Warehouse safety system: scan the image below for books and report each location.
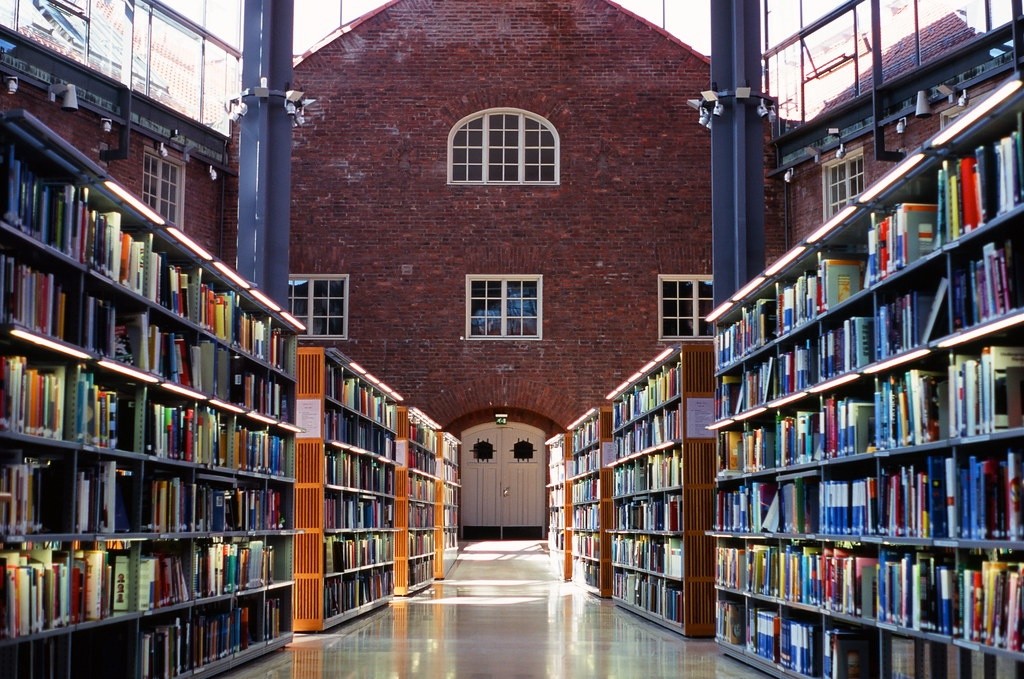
[0,154,460,678]
[548,132,1024,679]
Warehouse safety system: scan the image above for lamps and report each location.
[209,164,217,180]
[915,90,932,118]
[784,168,794,182]
[958,90,968,106]
[6,77,18,95]
[48,84,78,111]
[896,116,907,133]
[100,118,112,133]
[154,141,168,157]
[835,144,846,159]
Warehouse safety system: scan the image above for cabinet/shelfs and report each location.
[293,347,404,632]
[0,109,305,679]
[605,343,716,636]
[544,433,572,581]
[395,406,442,596]
[567,407,613,597]
[707,82,1023,679]
[435,432,462,578]
